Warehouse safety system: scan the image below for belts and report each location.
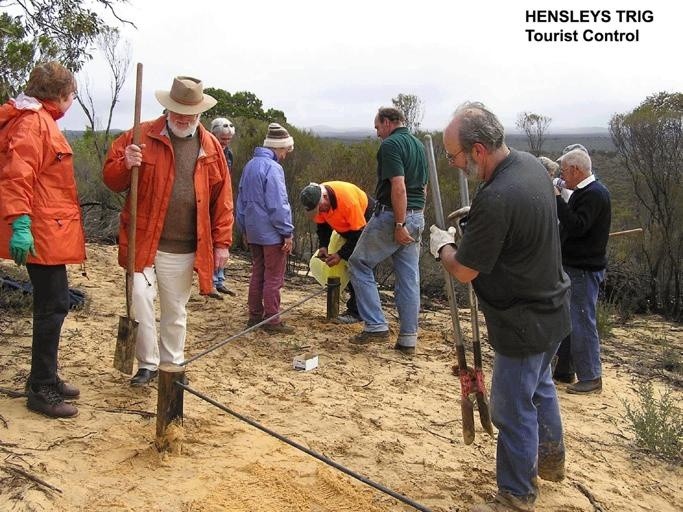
[381,206,422,212]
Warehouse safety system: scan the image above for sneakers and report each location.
[247,320,295,334]
[131,369,158,385]
[335,315,358,323]
[552,374,575,385]
[350,331,389,344]
[210,285,234,300]
[470,492,535,512]
[567,378,603,395]
[26,375,79,418]
[394,343,415,354]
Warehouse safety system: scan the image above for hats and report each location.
[156,76,217,115]
[263,123,294,148]
[301,186,320,218]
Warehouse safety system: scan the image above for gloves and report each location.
[429,225,457,260]
[9,215,37,267]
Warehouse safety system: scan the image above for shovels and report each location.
[113,63,143,375]
[424,134,494,446]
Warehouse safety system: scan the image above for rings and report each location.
[284,243,287,246]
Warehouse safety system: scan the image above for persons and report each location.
[429,100,573,511]
[347,106,429,356]
[300,179,373,324]
[200,117,236,300]
[235,123,297,334]
[537,143,611,396]
[0,61,86,419]
[102,77,235,386]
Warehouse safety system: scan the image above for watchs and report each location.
[394,221,406,228]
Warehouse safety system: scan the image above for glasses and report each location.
[446,149,462,165]
